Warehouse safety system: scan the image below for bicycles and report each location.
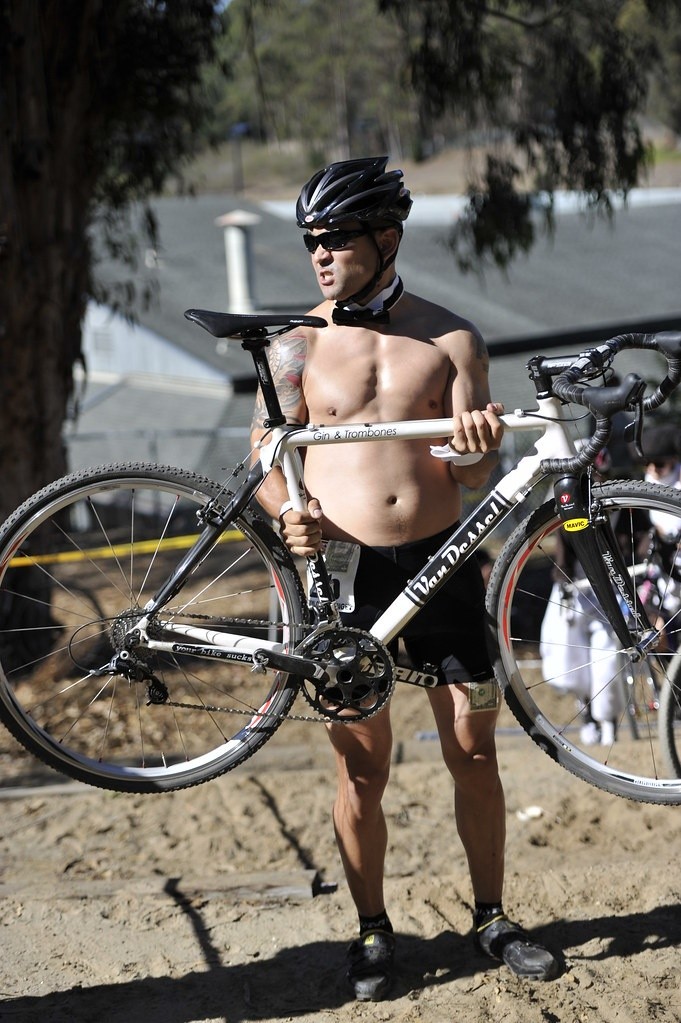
[1,310,681,805]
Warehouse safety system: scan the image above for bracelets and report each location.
[280,501,290,515]
[428,443,484,466]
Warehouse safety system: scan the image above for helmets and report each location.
[296,155,414,228]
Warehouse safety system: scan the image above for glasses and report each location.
[638,458,666,469]
[302,227,369,252]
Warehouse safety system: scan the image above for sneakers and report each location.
[469,911,559,982]
[345,928,400,1001]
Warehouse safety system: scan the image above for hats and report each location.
[626,421,681,457]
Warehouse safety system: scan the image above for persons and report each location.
[249,155,558,1002]
[539,457,681,746]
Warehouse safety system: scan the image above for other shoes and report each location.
[579,720,601,748]
[600,717,618,748]
